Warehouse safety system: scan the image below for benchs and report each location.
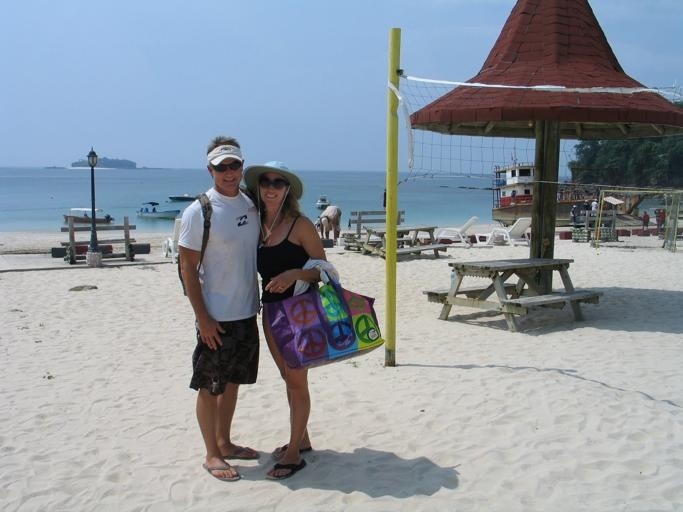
[384,243,448,254]
[505,291,607,332]
[423,283,515,319]
[356,237,413,255]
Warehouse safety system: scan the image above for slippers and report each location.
[202,462,241,481]
[223,445,261,461]
[271,442,312,460]
[265,459,307,480]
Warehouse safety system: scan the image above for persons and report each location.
[177,138,262,482]
[642,209,664,231]
[140,207,157,213]
[243,162,326,480]
[570,198,609,224]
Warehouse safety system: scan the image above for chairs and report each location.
[435,216,480,249]
[476,218,532,248]
[163,218,182,264]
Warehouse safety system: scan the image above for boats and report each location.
[575,213,615,226]
[64,207,113,223]
[316,194,330,209]
[73,158,137,169]
[135,202,180,218]
[168,196,197,200]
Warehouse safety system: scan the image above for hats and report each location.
[206,144,245,167]
[242,160,304,202]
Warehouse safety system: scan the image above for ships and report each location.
[492,161,647,226]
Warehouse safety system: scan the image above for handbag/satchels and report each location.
[266,268,385,369]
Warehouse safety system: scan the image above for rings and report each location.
[279,287,283,289]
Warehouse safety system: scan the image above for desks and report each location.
[362,226,439,257]
[438,259,585,333]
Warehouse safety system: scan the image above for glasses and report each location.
[210,160,242,172]
[257,174,290,190]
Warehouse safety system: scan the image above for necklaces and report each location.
[263,216,285,247]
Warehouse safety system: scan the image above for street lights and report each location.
[87,147,101,253]
